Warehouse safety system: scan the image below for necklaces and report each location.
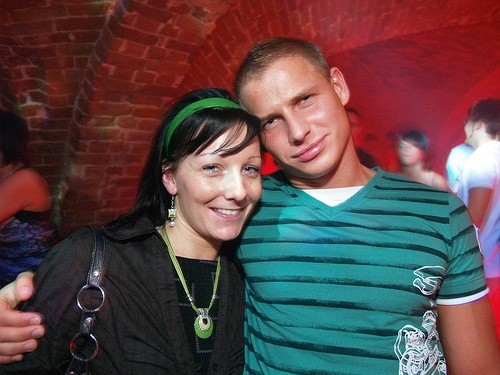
[161,227,222,339]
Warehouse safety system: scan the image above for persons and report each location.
[0,86,266,375]
[345,107,380,172]
[0,110,63,289]
[390,129,450,192]
[0,35,500,375]
[445,98,500,350]
